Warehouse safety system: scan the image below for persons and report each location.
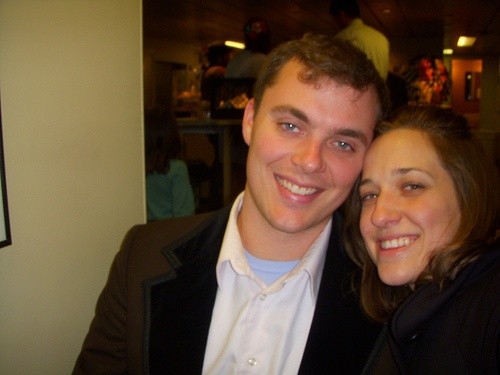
[200,41,233,117]
[145,106,194,221]
[70,36,386,375]
[342,106,500,375]
[221,17,272,119]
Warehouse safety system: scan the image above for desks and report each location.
[176,117,242,205]
